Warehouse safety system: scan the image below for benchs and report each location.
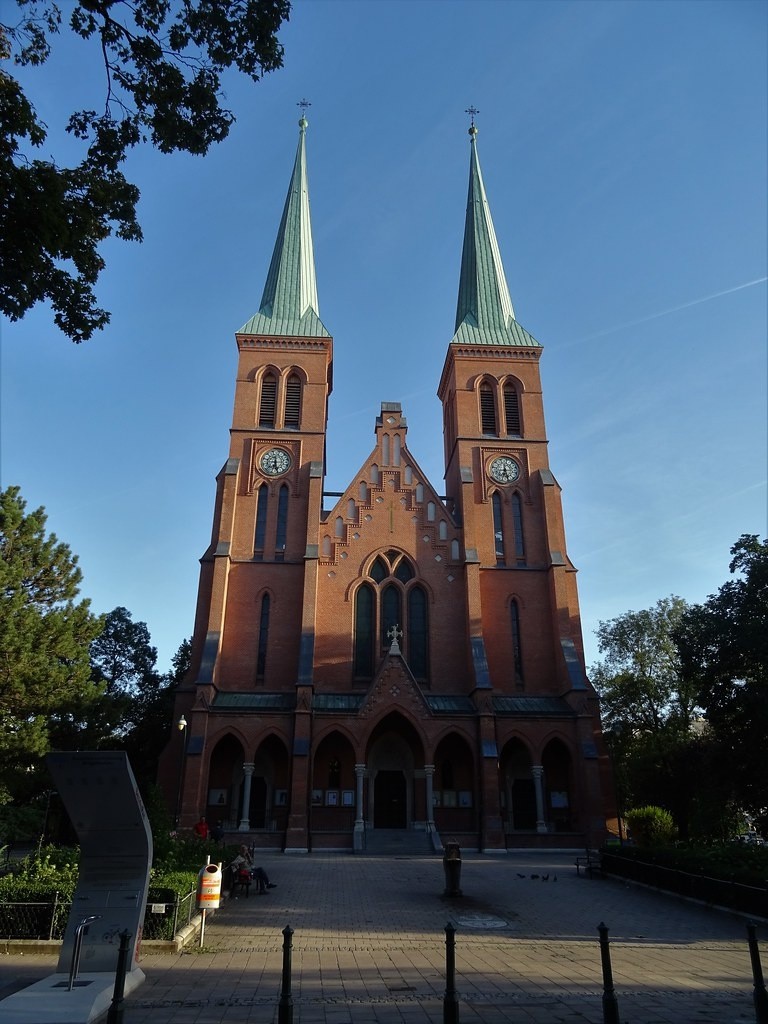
[229,866,258,898]
[575,853,610,880]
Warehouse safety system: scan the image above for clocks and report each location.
[485,451,525,486]
[255,443,296,479]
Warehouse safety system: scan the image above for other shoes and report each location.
[260,890,270,895]
[266,883,277,889]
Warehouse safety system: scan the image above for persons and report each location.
[231,845,277,896]
[193,815,225,846]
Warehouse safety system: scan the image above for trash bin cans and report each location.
[194,863,223,909]
